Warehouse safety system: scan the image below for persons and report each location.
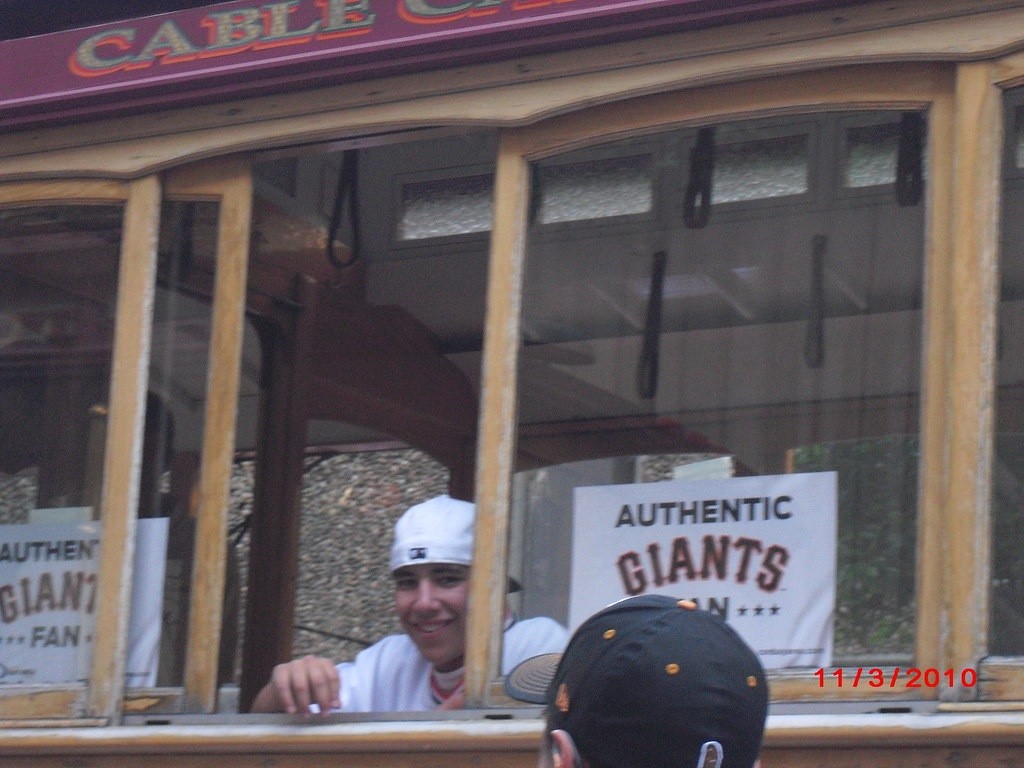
[255,493,570,718]
[504,594,770,768]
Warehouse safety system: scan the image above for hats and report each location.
[387,496,524,594]
[504,594,771,768]
